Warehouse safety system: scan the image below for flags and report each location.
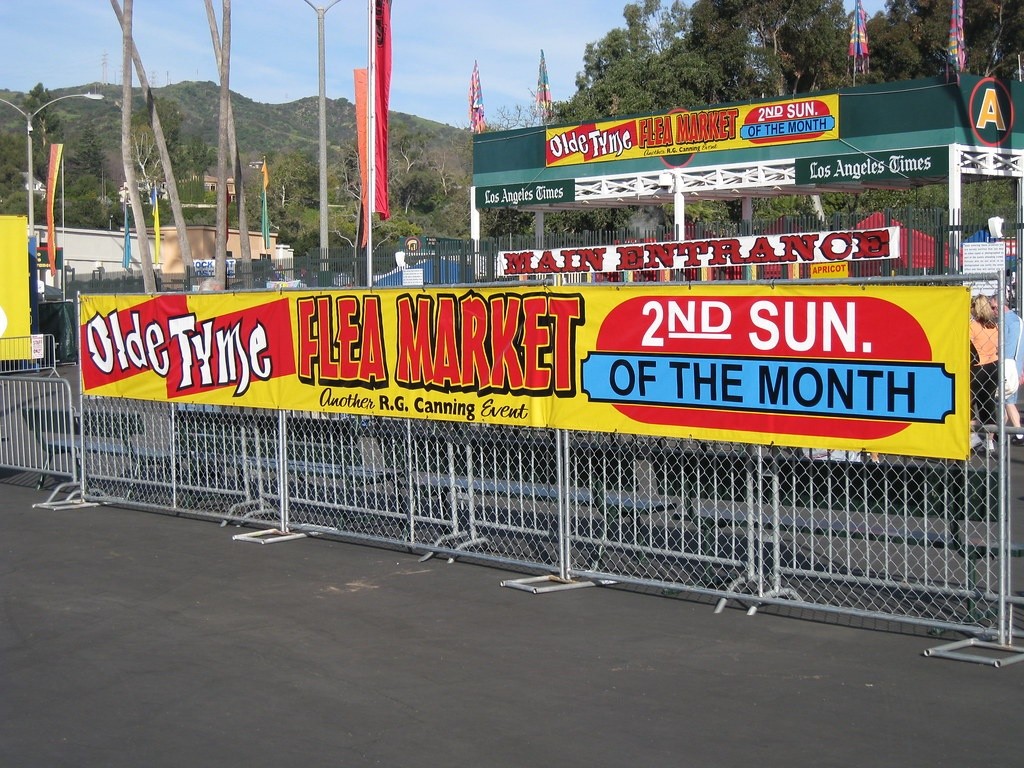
[468,65,485,133]
[946,1,965,87]
[261,161,270,249]
[215,187,231,242]
[372,0,393,221]
[849,1,869,75]
[152,189,160,265]
[536,49,551,119]
[47,144,64,277]
[354,69,369,248]
[122,199,130,270]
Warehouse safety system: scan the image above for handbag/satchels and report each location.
[970,340,980,365]
[994,359,1020,400]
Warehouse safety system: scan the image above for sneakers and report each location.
[973,442,995,453]
[1005,436,1024,446]
[970,432,982,449]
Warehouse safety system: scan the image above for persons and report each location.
[990,295,1024,446]
[970,294,999,453]
